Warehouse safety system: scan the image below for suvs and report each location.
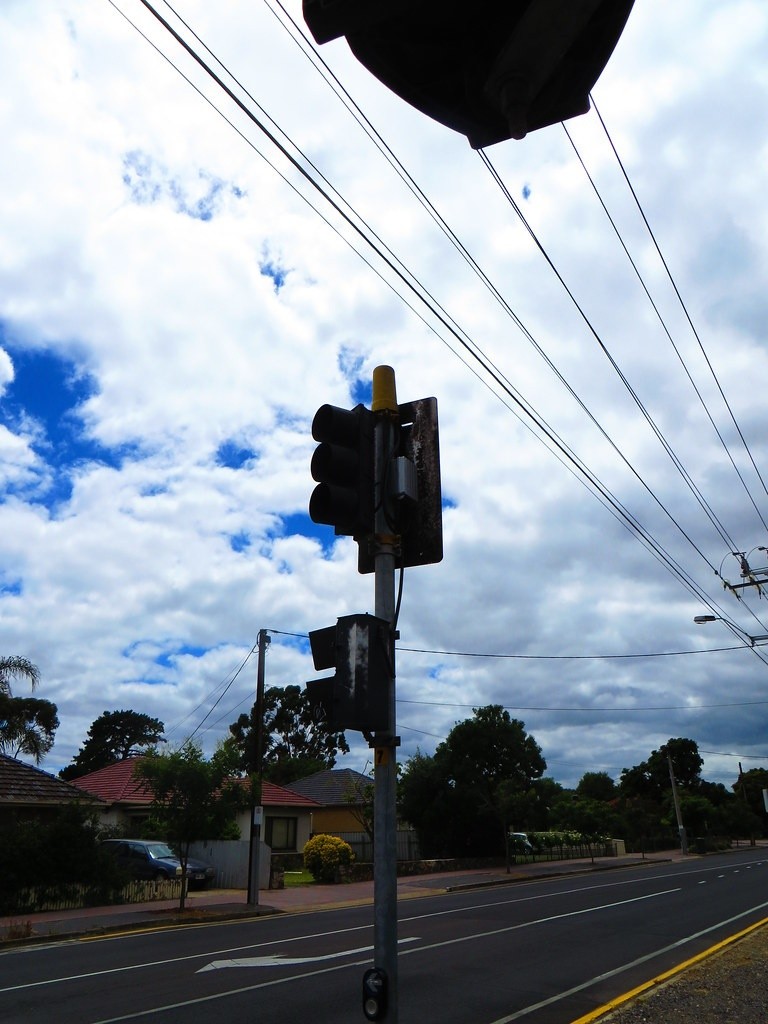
[106,837,217,893]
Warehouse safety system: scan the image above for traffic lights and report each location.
[299,401,369,534]
[304,612,392,736]
